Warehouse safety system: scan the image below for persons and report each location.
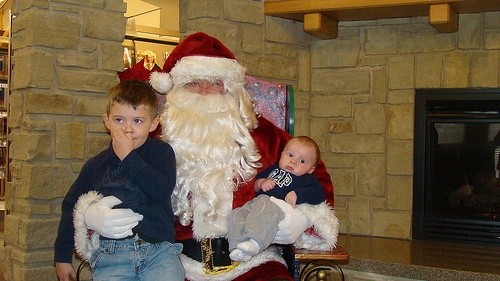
[227,135,320,262]
[73,31,339,281]
[53,80,186,279]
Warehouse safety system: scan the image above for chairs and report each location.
[75,243,351,281]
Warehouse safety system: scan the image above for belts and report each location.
[175,236,234,268]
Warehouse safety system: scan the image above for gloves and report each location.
[270,196,312,245]
[84,196,144,239]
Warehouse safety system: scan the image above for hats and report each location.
[149,31,246,95]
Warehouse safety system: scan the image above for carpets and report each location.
[299,257,500,280]
